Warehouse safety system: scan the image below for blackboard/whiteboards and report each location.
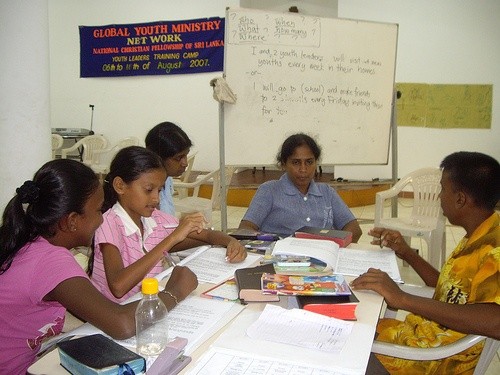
[222,4,399,166]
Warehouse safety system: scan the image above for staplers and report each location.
[146,335,192,375]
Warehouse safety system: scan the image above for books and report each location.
[56,226,403,375]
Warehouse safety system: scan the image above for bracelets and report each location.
[161,290,178,303]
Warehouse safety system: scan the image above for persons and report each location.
[91,146,247,304]
[0,159,198,375]
[238,134,363,243]
[349,150,500,375]
[145,122,206,252]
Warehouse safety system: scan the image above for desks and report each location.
[23,233,403,375]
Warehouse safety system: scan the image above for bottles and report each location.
[135,277,169,361]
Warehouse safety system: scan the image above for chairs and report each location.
[49,132,142,185]
[164,149,237,216]
[375,160,447,279]
[368,284,500,375]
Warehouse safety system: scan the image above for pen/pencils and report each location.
[162,224,179,228]
[163,250,177,267]
